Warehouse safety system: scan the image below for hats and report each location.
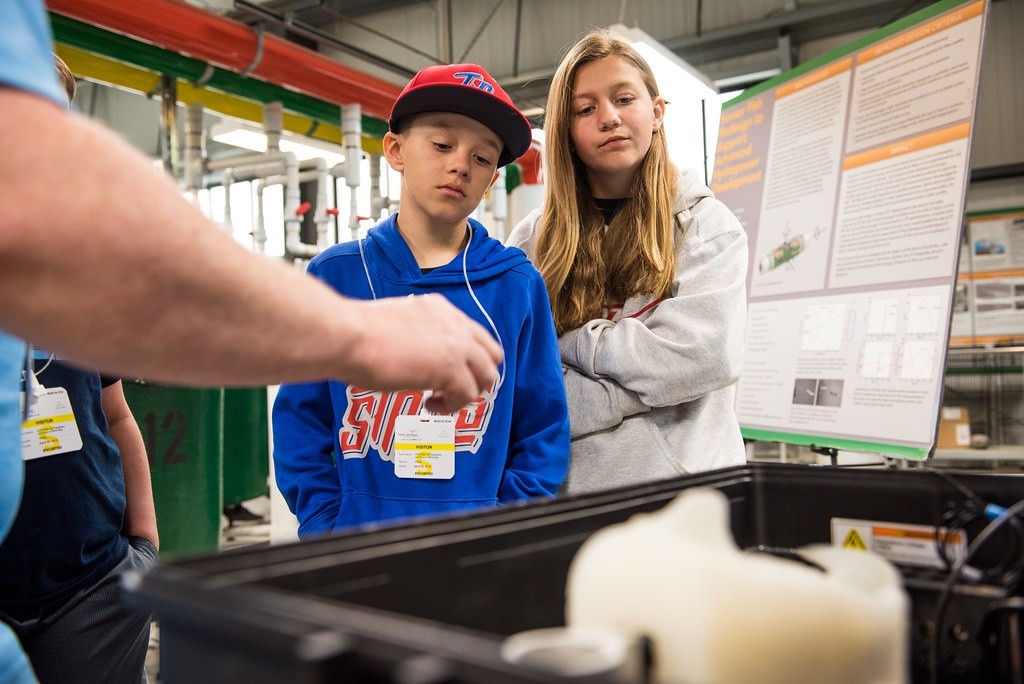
[389,63,532,168]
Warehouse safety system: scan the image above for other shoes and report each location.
[224,505,264,525]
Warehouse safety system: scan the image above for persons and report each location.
[0,0,502,684]
[272,62,571,539]
[503,35,748,492]
[0,343,159,684]
